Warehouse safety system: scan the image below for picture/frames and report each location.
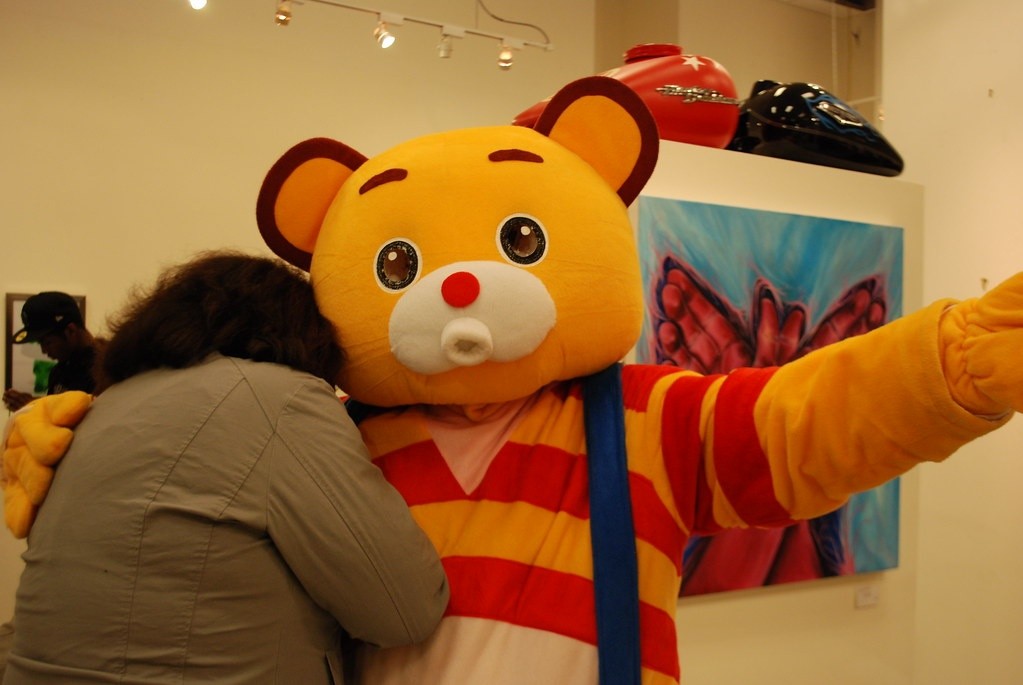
[4,292,87,399]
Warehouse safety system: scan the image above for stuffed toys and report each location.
[1,72,1023,685]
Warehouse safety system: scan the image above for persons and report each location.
[1,252,455,685]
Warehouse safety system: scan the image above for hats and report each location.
[11,291,81,344]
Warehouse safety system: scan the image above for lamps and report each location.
[435,25,466,58]
[272,0,305,27]
[372,12,404,49]
[496,38,524,71]
